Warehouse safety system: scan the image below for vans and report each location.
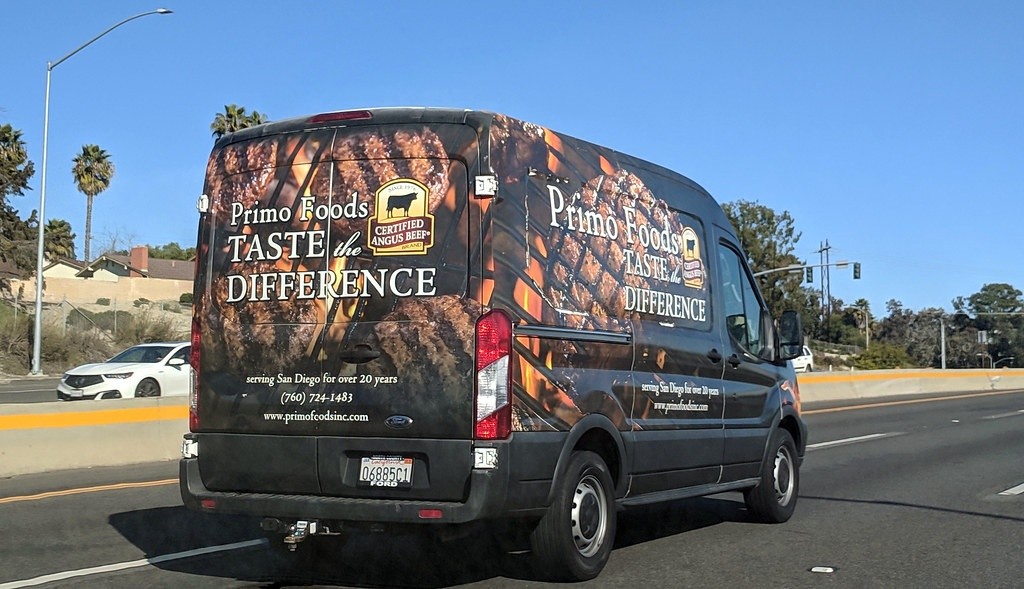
[176,105,810,583]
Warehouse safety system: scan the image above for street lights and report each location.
[993,357,1014,369]
[976,353,992,369]
[841,305,869,353]
[29,9,174,377]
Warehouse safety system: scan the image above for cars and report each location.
[789,345,814,374]
[56,341,193,402]
